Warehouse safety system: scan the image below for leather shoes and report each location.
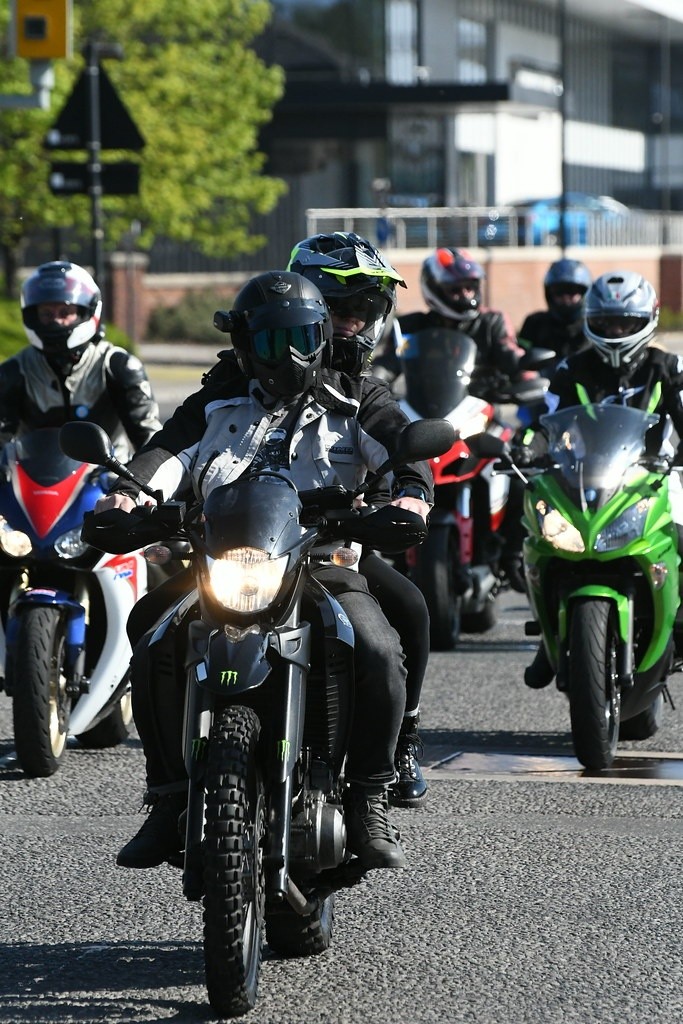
[388,734,428,808]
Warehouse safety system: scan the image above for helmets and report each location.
[582,271,659,369]
[230,272,333,397]
[544,259,592,323]
[20,261,102,356]
[285,231,407,379]
[420,247,483,321]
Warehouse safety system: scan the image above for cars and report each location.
[482,192,635,246]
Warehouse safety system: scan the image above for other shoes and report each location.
[524,640,554,689]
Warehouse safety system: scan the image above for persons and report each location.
[476,258,594,566]
[504,269,683,690]
[0,262,185,694]
[370,246,532,595]
[96,233,433,875]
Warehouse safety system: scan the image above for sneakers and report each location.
[345,788,405,868]
[116,792,187,869]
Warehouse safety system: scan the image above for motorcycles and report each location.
[389,334,561,652]
[1,407,133,779]
[464,403,683,770]
[59,414,455,1010]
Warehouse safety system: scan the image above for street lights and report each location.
[81,40,126,295]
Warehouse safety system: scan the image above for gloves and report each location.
[511,447,535,464]
